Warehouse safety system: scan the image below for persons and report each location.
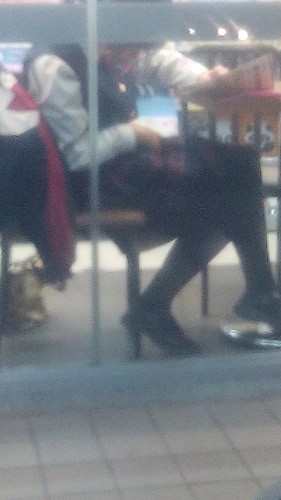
[27,31,280,351]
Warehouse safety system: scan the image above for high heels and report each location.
[121,299,203,359]
[232,291,281,330]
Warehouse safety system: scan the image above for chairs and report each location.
[0,70,209,361]
